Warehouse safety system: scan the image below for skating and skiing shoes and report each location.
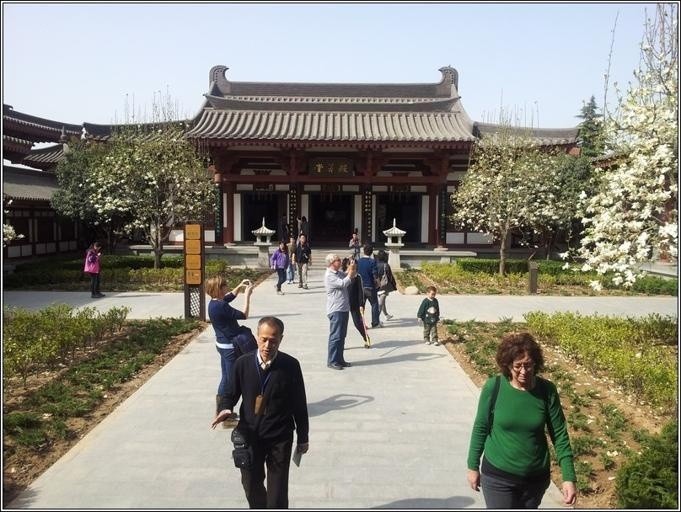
[365,313,393,348]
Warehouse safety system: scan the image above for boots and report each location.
[216,394,237,418]
[222,412,239,428]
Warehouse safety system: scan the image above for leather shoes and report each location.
[327,361,342,370]
[339,361,352,367]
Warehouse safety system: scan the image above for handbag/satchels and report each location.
[229,424,253,469]
[230,326,258,358]
[380,265,395,292]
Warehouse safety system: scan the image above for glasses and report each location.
[512,362,533,373]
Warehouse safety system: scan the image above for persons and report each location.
[210,317,309,509]
[417,286,440,346]
[468,333,577,509]
[83,240,105,298]
[270,215,396,370]
[204,275,253,430]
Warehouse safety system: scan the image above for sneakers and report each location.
[274,280,308,295]
[425,341,431,345]
[91,294,100,298]
[433,341,439,346]
[95,292,104,297]
